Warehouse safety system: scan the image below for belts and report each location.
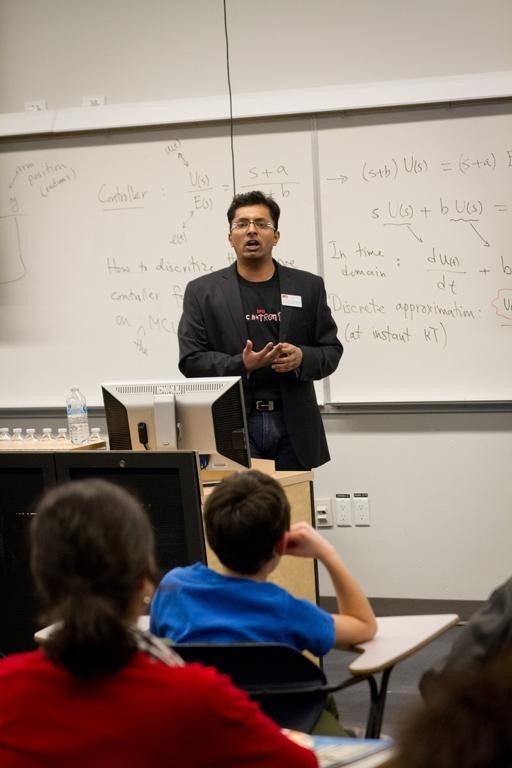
[252,398,283,410]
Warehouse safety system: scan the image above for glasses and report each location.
[231,218,276,231]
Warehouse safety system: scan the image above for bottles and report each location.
[0,427,67,441]
[66,385,89,445]
[90,428,102,442]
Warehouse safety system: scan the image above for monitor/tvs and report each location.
[102,376,252,487]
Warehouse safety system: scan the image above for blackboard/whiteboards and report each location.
[0,95,512,419]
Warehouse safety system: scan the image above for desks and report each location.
[293,727,401,767]
[33,606,461,739]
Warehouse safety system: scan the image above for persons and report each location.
[150,470,378,658]
[177,190,344,472]
[1,478,323,766]
[375,580,512,768]
